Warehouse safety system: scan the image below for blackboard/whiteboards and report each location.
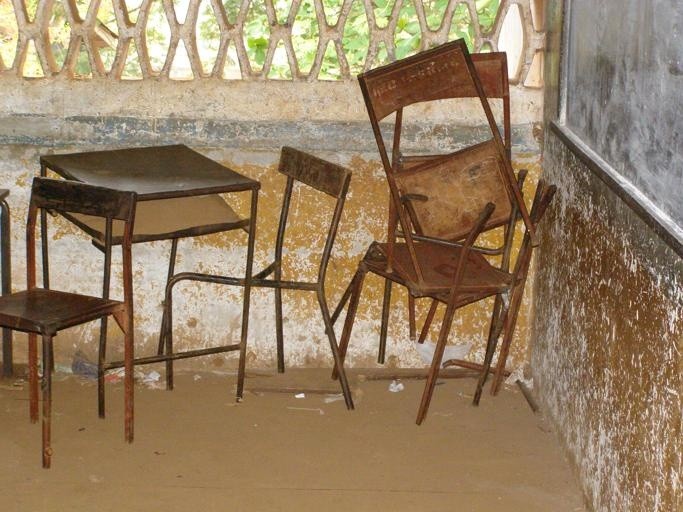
[550,1,683,260]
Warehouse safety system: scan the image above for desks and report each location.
[39,143,261,414]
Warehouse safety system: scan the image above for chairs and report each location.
[0,176,136,468]
[167,146,354,411]
